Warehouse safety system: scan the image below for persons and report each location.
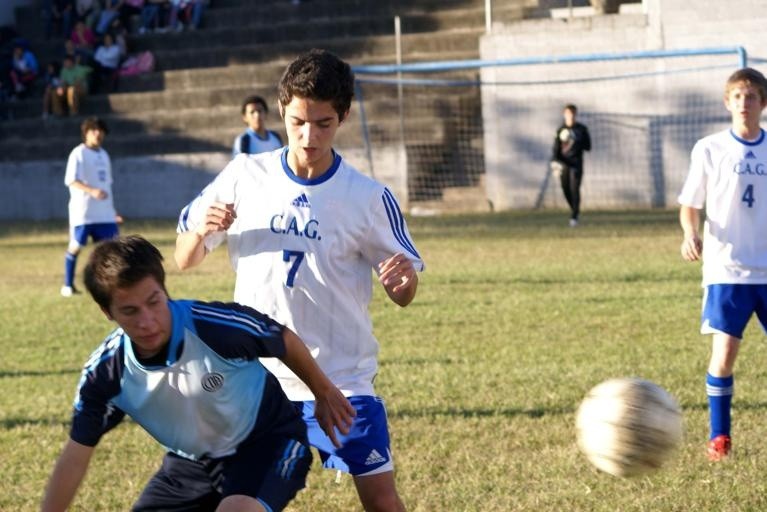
[175,49,425,512]
[677,68,767,463]
[549,105,591,227]
[232,95,284,160]
[0,0,202,128]
[39,234,357,512]
[61,117,123,298]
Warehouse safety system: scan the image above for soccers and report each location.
[576,381,681,476]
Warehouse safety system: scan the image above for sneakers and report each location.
[707,433,731,464]
[61,285,79,297]
